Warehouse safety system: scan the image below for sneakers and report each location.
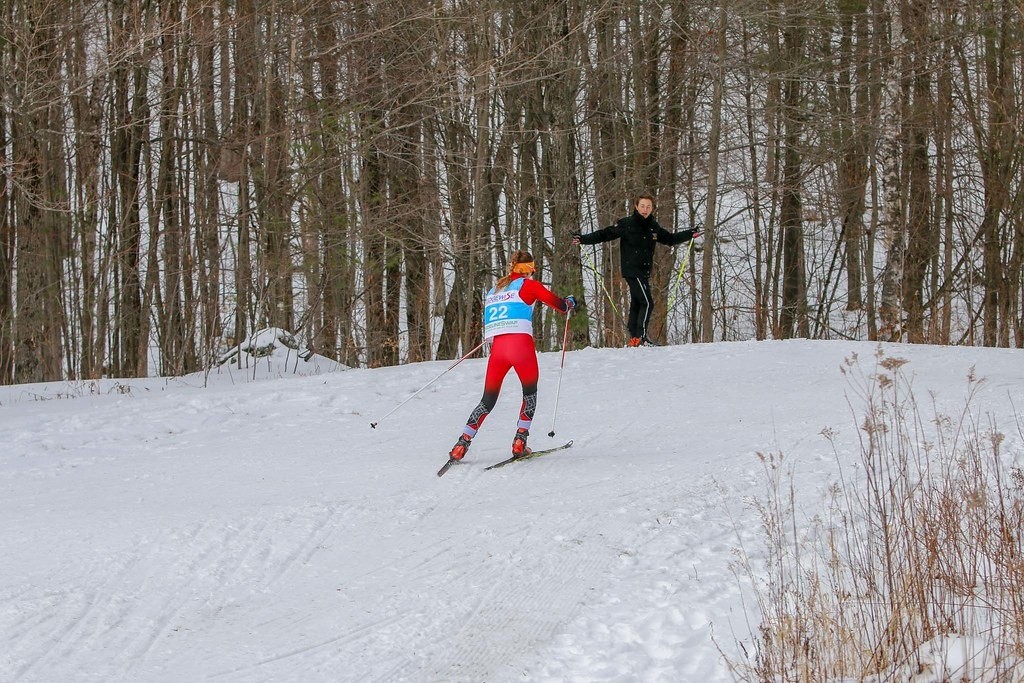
[450,433,470,461]
[512,428,532,455]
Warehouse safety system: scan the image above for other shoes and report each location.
[629,337,653,347]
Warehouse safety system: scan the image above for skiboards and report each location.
[437,441,573,477]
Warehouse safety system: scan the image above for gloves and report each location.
[564,294,578,309]
[691,227,700,238]
[573,234,580,245]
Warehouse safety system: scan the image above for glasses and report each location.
[527,264,536,276]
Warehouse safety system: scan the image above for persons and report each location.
[449,250,576,461]
[572,195,701,348]
